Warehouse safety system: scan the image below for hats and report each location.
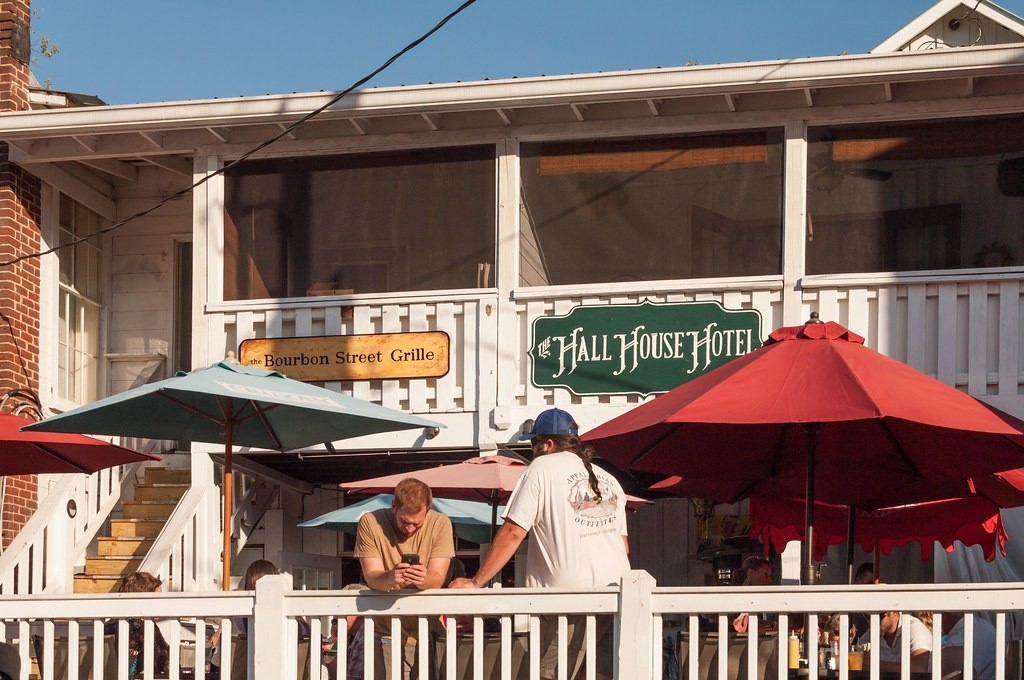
[518,407,579,441]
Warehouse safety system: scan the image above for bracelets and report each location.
[472,576,483,589]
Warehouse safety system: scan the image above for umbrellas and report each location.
[0,352,448,597]
[299,311,1024,587]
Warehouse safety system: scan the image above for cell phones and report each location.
[402,554,420,565]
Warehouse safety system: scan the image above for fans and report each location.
[766,141,894,182]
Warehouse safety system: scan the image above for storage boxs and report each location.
[307,281,354,295]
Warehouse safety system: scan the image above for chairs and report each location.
[1005,639,1024,680]
[674,629,778,680]
[940,645,964,680]
[32,633,530,680]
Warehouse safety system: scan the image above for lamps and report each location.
[521,419,534,435]
[425,426,440,440]
[814,171,846,193]
[691,497,716,519]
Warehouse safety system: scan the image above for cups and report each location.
[830,640,839,657]
[852,645,862,653]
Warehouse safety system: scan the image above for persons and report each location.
[99,407,1007,680]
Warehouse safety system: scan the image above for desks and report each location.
[787,667,931,680]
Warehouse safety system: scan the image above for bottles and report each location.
[789,630,799,669]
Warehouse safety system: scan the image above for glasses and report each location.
[833,629,854,637]
[530,436,560,447]
[865,613,888,622]
